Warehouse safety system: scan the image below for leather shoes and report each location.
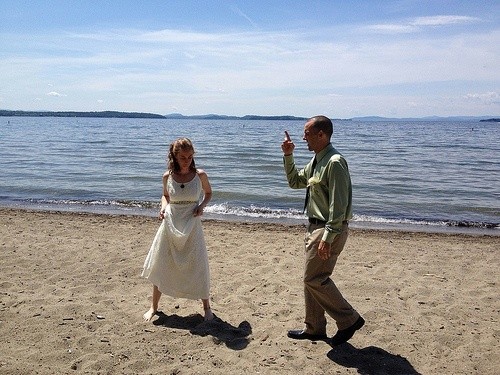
[288,330,327,339]
[331,315,365,343]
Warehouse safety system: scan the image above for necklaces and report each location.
[177,169,191,189]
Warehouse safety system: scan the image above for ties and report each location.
[304,156,317,211]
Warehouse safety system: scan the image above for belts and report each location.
[309,217,347,225]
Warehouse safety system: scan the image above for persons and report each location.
[280,115,365,345]
[143,136,214,322]
